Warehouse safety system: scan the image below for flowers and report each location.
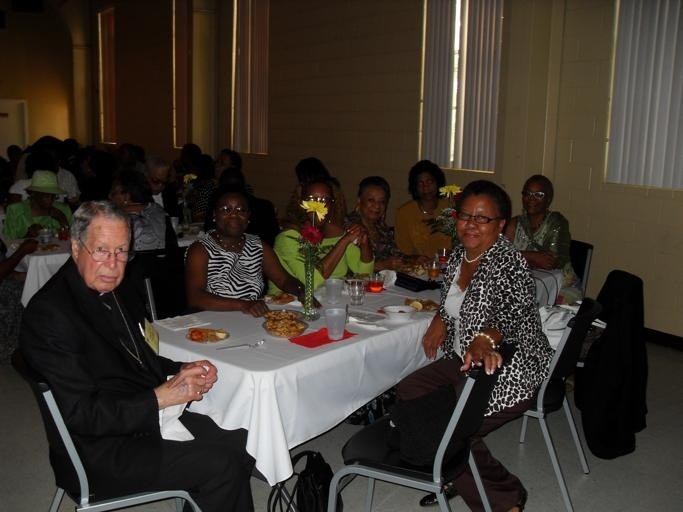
[286,201,328,273]
[435,185,463,239]
[178,173,198,206]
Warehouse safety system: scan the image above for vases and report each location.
[179,208,192,233]
[304,265,319,320]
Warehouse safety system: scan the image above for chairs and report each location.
[520,297,602,512]
[570,239,593,296]
[327,346,515,512]
[11,351,201,512]
[142,265,187,322]
[575,270,643,411]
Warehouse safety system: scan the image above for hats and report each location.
[24,170,65,195]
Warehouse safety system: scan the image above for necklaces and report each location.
[215,233,245,251]
[111,289,147,371]
[419,200,433,215]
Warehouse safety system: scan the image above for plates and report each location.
[262,320,309,338]
[264,308,307,321]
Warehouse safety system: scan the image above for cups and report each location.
[40,227,51,246]
[368,272,386,292]
[346,279,366,305]
[171,216,179,232]
[324,308,347,341]
[325,278,343,305]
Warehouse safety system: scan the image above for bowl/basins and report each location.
[382,304,417,322]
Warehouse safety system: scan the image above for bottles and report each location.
[429,247,448,279]
[58,224,68,241]
[181,206,192,231]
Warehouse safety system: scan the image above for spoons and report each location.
[216,339,265,351]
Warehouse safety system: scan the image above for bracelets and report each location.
[473,331,497,351]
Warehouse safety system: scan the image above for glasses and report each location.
[458,212,500,224]
[78,236,135,263]
[523,191,545,200]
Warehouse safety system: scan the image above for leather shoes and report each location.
[420,487,456,506]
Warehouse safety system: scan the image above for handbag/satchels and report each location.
[297,452,343,512]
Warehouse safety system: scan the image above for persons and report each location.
[0,155,40,367]
[263,178,376,295]
[282,155,349,229]
[391,158,462,266]
[378,179,556,512]
[16,197,254,512]
[183,182,324,318]
[0,134,280,279]
[503,174,584,349]
[344,175,432,275]
